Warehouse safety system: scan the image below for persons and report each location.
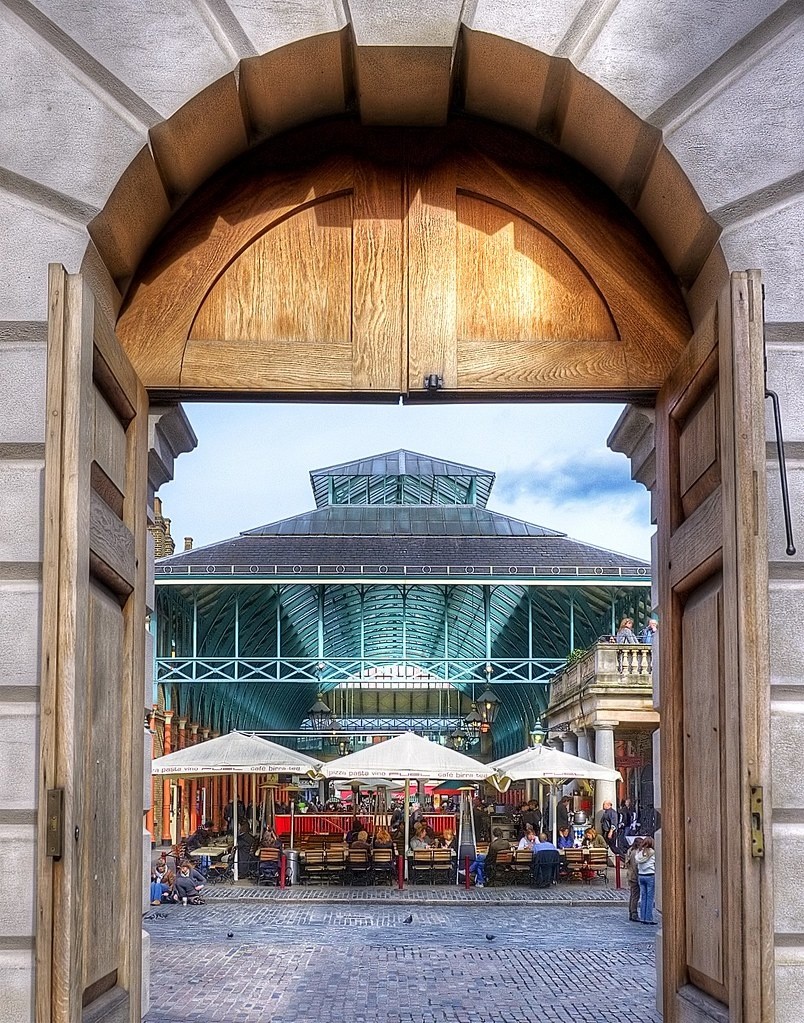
[184,795,637,888]
[150,856,207,906]
[637,619,657,674]
[616,617,642,674]
[634,836,658,926]
[625,837,643,922]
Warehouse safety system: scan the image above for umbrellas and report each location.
[319,728,499,881]
[152,730,325,881]
[336,779,405,823]
[431,780,477,795]
[484,743,624,849]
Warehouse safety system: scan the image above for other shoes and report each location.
[632,918,642,922]
[475,881,484,888]
[150,900,160,905]
[629,918,632,920]
[458,868,471,876]
[641,921,658,925]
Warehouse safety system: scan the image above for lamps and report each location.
[308,692,351,757]
[528,716,549,746]
[450,679,502,751]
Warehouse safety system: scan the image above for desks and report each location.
[189,833,233,883]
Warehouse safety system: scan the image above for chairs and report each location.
[208,845,238,884]
[212,848,236,878]
[256,828,610,885]
[171,841,187,875]
